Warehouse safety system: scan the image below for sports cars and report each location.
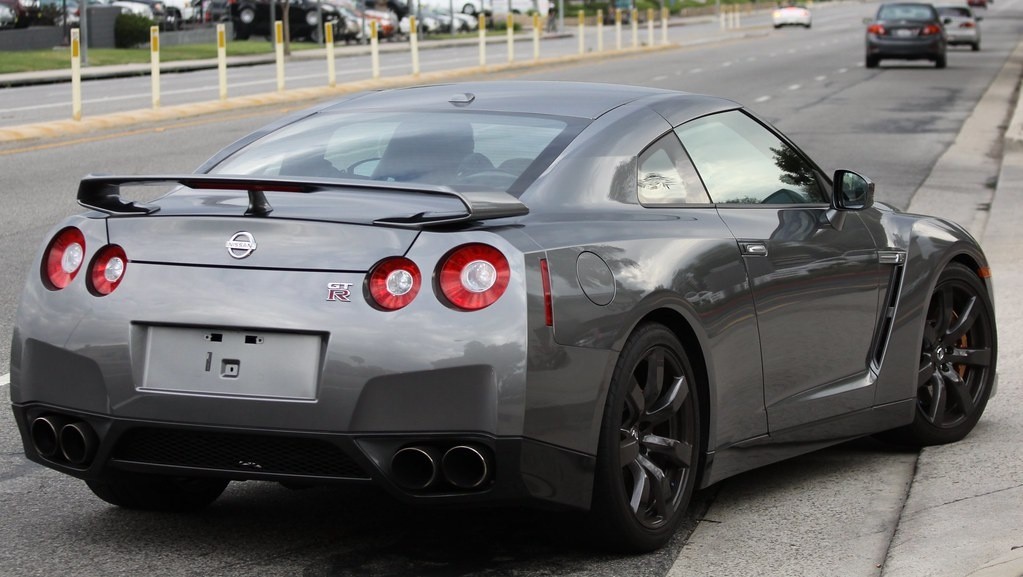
[8,83,1005,553]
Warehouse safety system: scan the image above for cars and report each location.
[0,0,644,41]
[933,4,983,51]
[968,0,986,7]
[863,3,948,70]
[773,5,812,27]
